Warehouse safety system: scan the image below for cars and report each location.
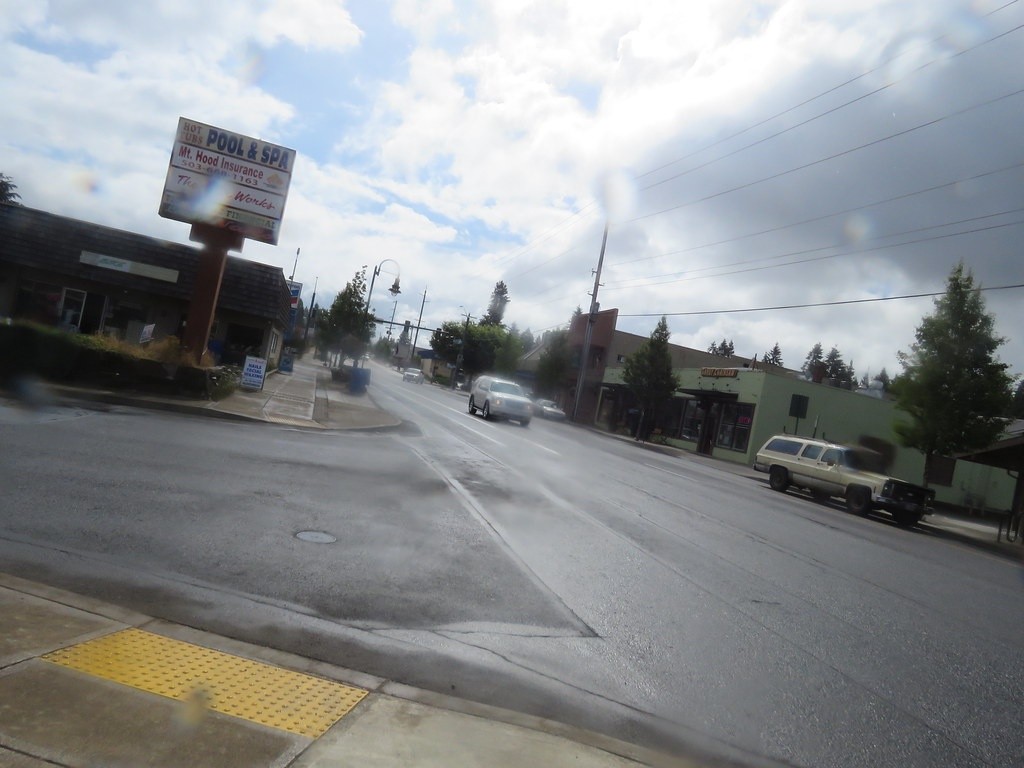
[403,368,425,384]
[533,399,567,422]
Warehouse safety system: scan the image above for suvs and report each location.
[468,375,534,427]
[754,432,935,526]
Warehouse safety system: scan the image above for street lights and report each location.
[451,305,471,392]
[355,257,404,360]
